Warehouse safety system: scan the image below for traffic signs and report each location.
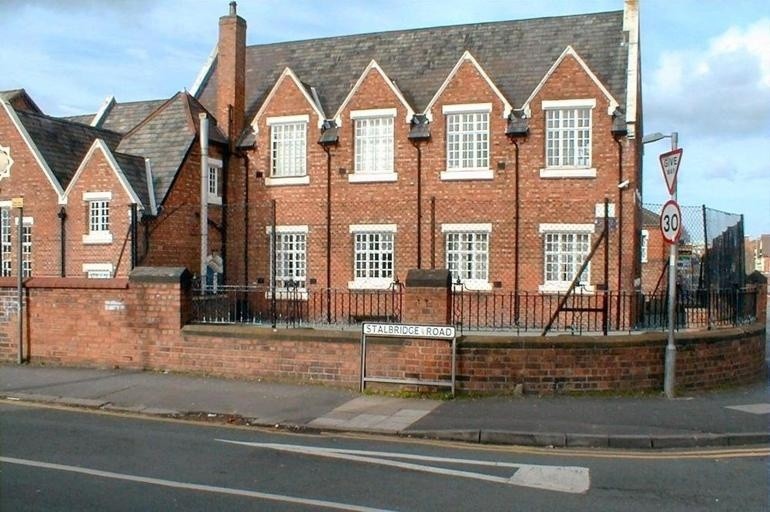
[661,201,682,243]
[361,322,456,340]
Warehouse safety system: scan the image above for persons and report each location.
[206,249,224,296]
